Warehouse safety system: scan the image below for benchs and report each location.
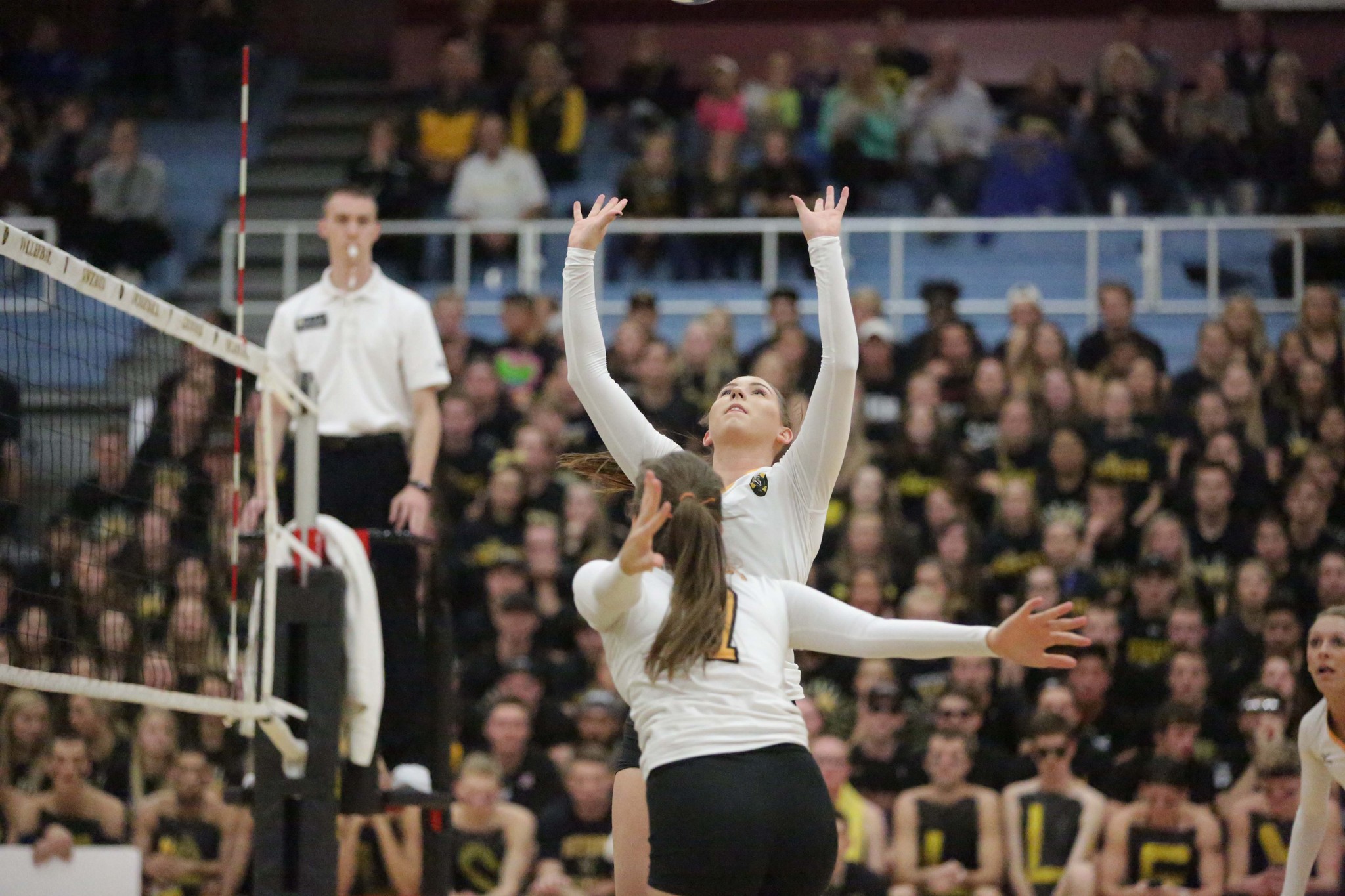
[0,0,1345,390]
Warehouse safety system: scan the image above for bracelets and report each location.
[409,479,434,494]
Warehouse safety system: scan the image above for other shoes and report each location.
[390,763,433,795]
[242,741,307,788]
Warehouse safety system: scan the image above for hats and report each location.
[869,681,900,699]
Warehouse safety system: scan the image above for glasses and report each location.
[1036,745,1067,760]
[939,706,972,721]
[1242,697,1283,714]
[867,704,902,716]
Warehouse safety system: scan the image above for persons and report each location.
[571,450,1091,896]
[239,186,453,795]
[0,0,1345,896]
[563,183,907,896]
